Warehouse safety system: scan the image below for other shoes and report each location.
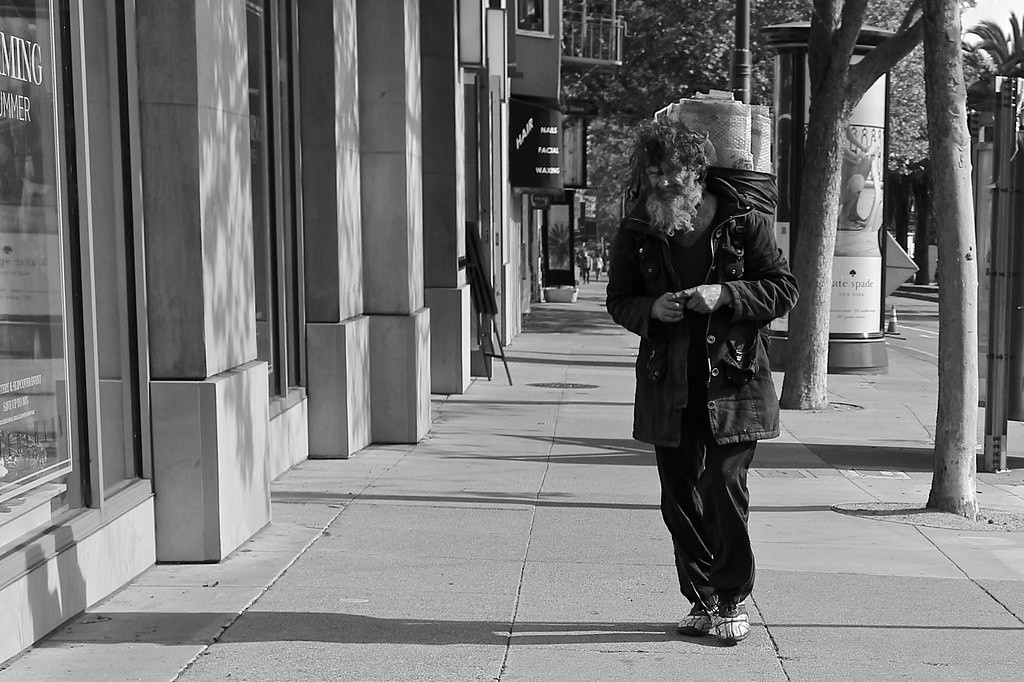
[676,593,722,636]
[714,599,751,643]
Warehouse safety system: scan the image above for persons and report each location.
[576,251,611,284]
[606,115,800,643]
[838,124,873,228]
